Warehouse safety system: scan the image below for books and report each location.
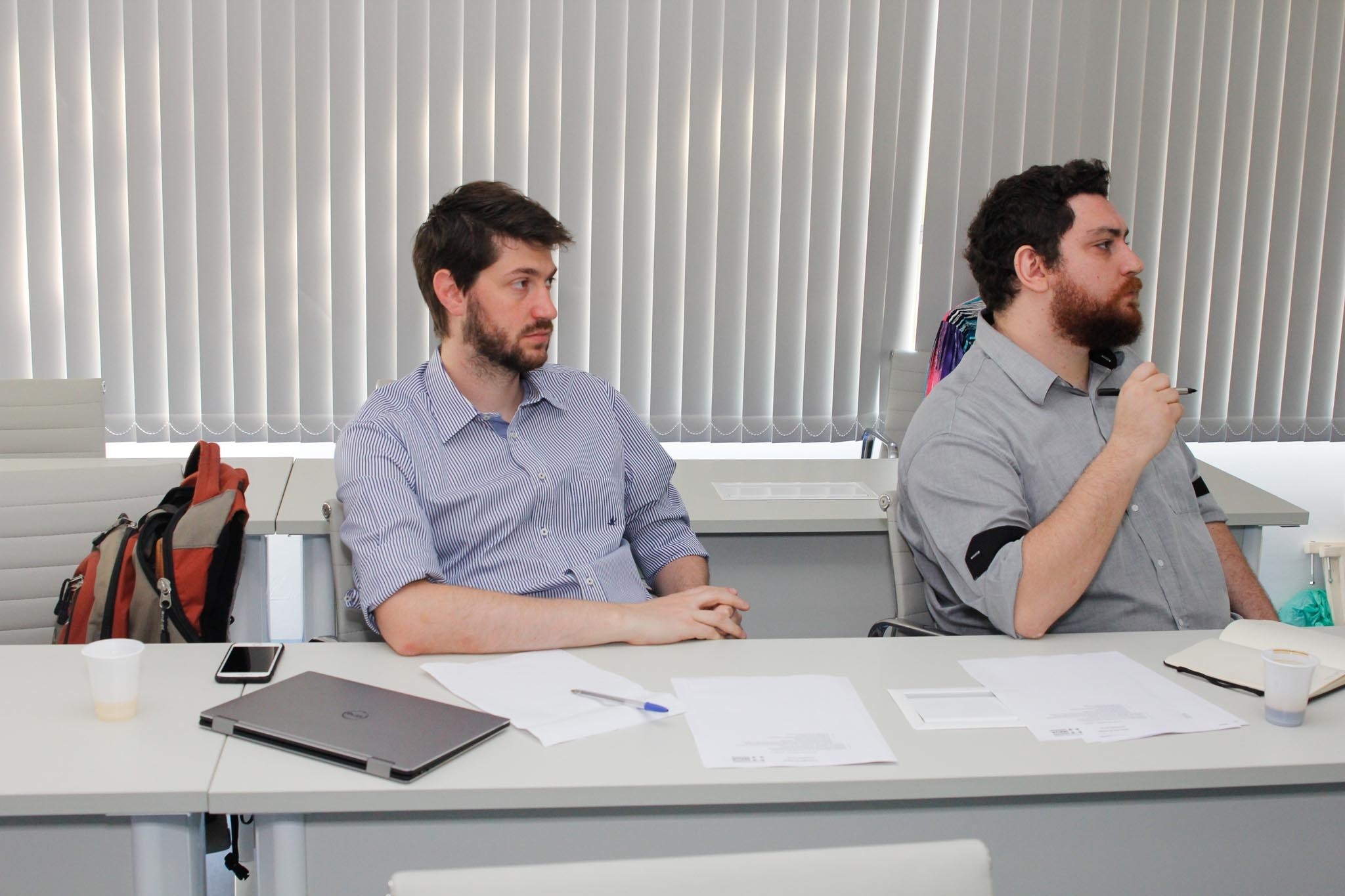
[1164,620,1345,702]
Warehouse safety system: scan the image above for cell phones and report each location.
[215,643,284,684]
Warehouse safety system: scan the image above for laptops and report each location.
[197,671,511,783]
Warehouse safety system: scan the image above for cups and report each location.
[1262,649,1320,727]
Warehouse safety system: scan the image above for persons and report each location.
[336,180,749,657]
[894,160,1279,639]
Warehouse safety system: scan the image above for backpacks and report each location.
[47,440,250,650]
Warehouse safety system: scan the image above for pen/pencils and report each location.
[1097,388,1197,396]
[571,689,668,712]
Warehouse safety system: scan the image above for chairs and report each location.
[860,350,933,459]
[865,490,953,637]
[305,498,382,645]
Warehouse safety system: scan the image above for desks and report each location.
[275,456,1307,642]
[0,628,1345,895]
